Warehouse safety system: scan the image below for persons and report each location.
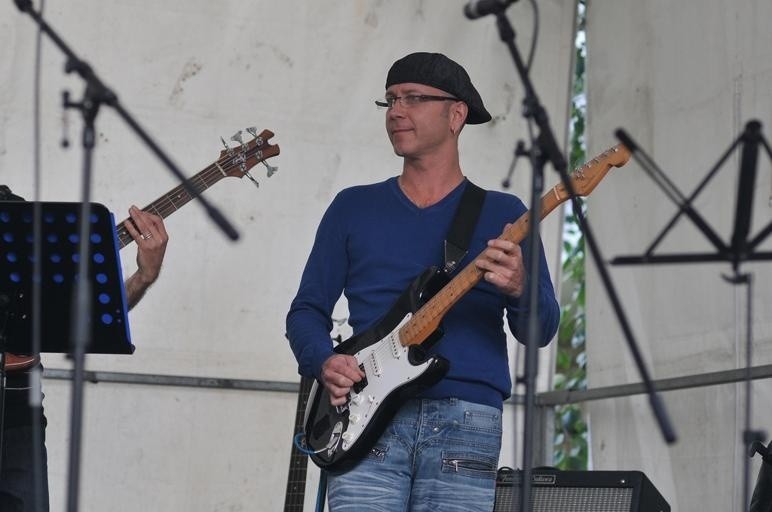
[287,52,561,512]
[0,182,168,511]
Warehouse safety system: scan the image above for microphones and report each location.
[464,0,512,19]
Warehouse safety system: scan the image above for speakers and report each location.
[493,467,671,512]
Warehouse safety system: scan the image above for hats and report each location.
[386,52,491,124]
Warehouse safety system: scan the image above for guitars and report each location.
[0,126,281,372]
[303,143,633,477]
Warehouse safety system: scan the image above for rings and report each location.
[144,233,152,239]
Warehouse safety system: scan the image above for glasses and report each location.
[375,94,455,109]
